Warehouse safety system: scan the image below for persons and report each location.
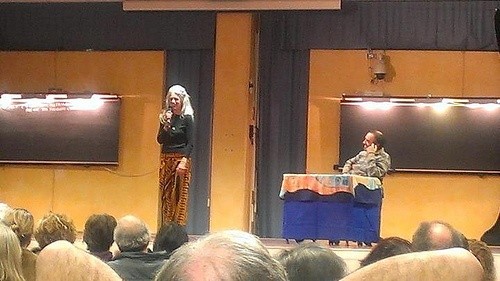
[156,84,194,228]
[0,209,494,281]
[341,130,391,183]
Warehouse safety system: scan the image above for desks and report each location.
[278,172,382,247]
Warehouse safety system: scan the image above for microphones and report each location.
[167,106,172,123]
